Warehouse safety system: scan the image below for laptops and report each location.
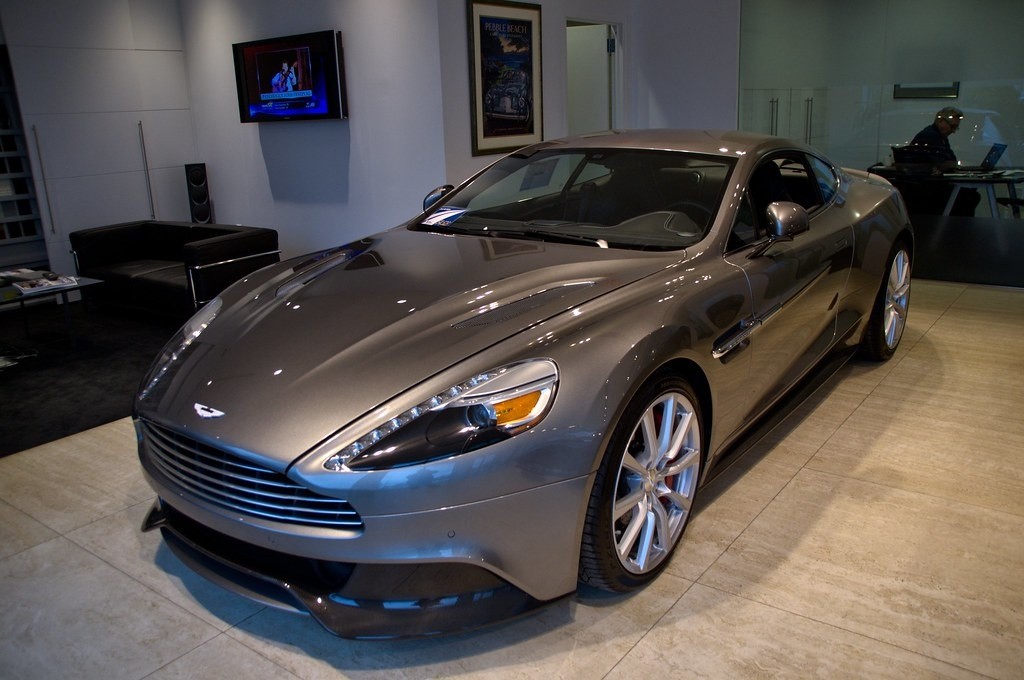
[956,144,1008,171]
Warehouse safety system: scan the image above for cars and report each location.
[132,126,914,642]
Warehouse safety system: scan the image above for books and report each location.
[11,275,79,295]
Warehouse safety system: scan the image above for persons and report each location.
[271,61,297,93]
[907,106,980,212]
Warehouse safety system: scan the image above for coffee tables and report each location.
[0,268,104,346]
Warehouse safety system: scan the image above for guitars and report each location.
[272,61,297,93]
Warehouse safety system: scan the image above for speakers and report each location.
[185,163,213,225]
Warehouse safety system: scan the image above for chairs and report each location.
[892,145,947,163]
[713,161,795,225]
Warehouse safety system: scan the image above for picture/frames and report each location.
[466,1,544,157]
[892,81,960,99]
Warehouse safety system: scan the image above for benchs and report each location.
[630,170,818,228]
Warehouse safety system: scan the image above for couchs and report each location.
[68,219,280,318]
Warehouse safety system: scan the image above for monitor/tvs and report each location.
[232,29,348,123]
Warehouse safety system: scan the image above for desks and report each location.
[928,167,1023,256]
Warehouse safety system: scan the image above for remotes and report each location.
[43,272,58,281]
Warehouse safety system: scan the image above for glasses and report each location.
[938,117,960,130]
